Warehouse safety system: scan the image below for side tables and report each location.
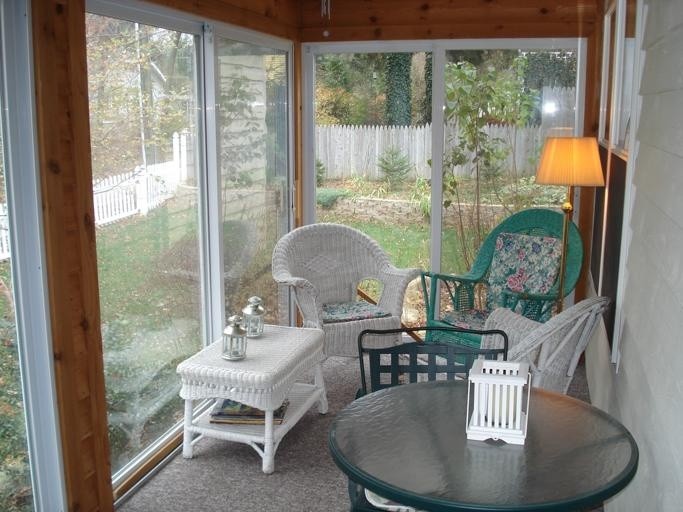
[174,324,327,475]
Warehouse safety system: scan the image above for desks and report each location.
[328,380,638,512]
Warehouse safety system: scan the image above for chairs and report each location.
[355,325,510,512]
[272,221,422,359]
[421,208,585,365]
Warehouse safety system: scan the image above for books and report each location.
[208,398,290,425]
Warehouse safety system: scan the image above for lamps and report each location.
[533,135,606,315]
